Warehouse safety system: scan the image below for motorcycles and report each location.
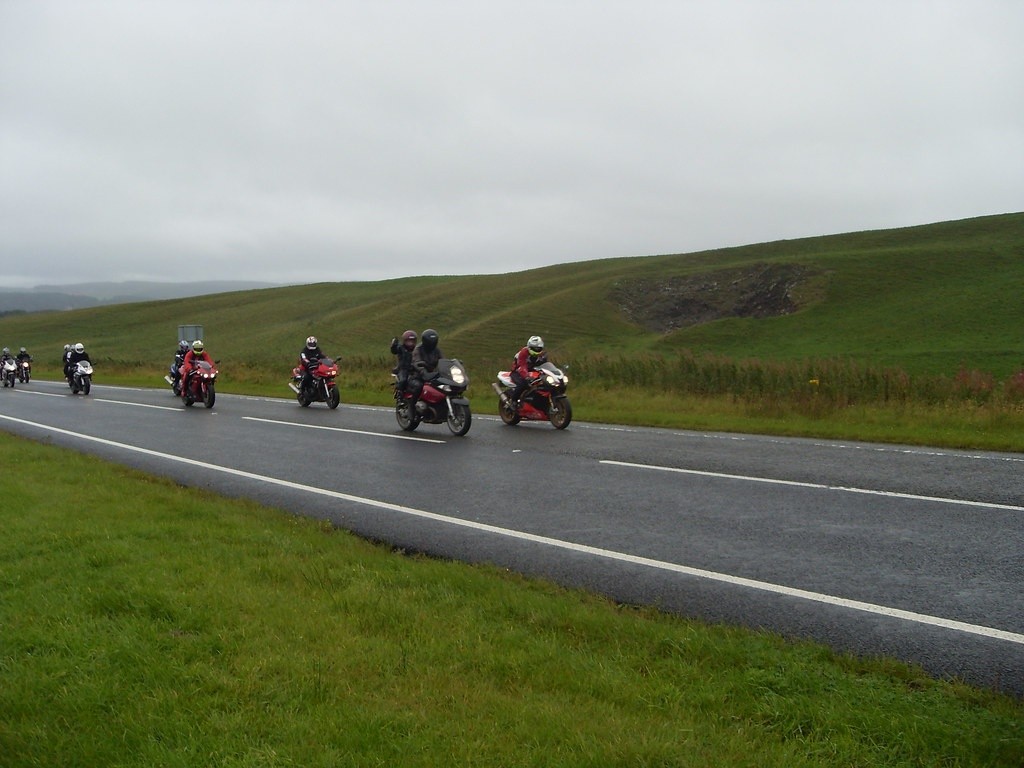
[177,359,221,409]
[164,354,185,396]
[15,355,34,384]
[390,357,474,437]
[289,353,342,409]
[0,357,21,387]
[492,361,577,430]
[63,360,99,395]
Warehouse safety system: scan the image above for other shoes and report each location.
[397,390,403,399]
[509,398,516,411]
[407,404,415,421]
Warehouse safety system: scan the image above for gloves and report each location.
[391,337,399,345]
[524,377,534,387]
[307,362,315,371]
[178,361,182,367]
[189,370,195,377]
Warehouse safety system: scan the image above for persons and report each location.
[63,343,92,380]
[171,340,191,387]
[406,329,443,421]
[15,346,34,378]
[298,335,327,401]
[0,347,17,382]
[181,339,217,398]
[509,335,547,411]
[391,329,417,406]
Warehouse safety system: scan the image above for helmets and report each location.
[75,343,84,354]
[2,348,9,356]
[64,344,71,352]
[421,328,438,350]
[402,330,417,350]
[527,335,544,358]
[306,336,317,349]
[179,341,188,352]
[20,347,26,355]
[71,344,75,353]
[192,339,204,355]
